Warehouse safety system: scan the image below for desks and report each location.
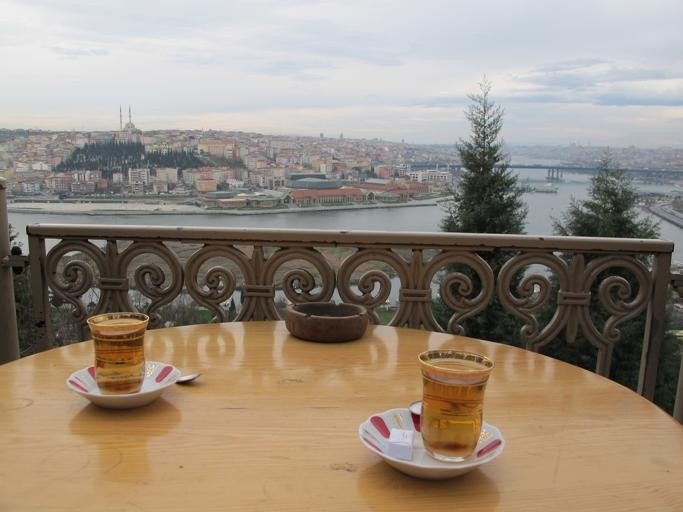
[0,319,683,512]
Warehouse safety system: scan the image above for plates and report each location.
[358,400,507,481]
[65,359,182,412]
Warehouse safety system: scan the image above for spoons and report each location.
[174,372,201,386]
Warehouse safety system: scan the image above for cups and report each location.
[415,347,496,465]
[86,311,150,394]
